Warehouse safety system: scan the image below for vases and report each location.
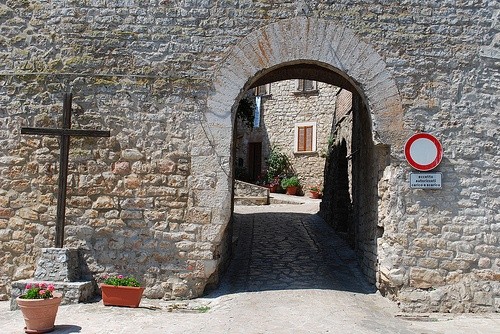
[270,186,277,192]
[16,292,63,333]
[309,189,320,198]
[101,284,145,308]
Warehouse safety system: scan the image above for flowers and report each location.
[263,179,279,188]
[102,272,141,287]
[24,282,54,299]
[306,179,321,191]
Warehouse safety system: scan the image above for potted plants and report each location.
[265,151,288,184]
[281,176,301,194]
